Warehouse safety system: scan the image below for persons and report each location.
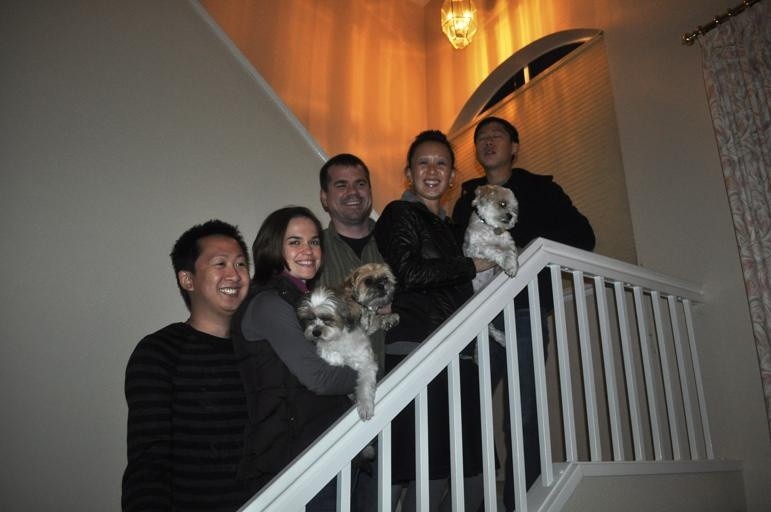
[227,206,379,511]
[371,125,511,511]
[122,218,274,511]
[304,154,420,512]
[447,116,595,512]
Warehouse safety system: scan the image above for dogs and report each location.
[295,285,379,460]
[462,184,520,366]
[343,262,400,336]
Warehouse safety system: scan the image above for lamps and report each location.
[440,0,480,50]
